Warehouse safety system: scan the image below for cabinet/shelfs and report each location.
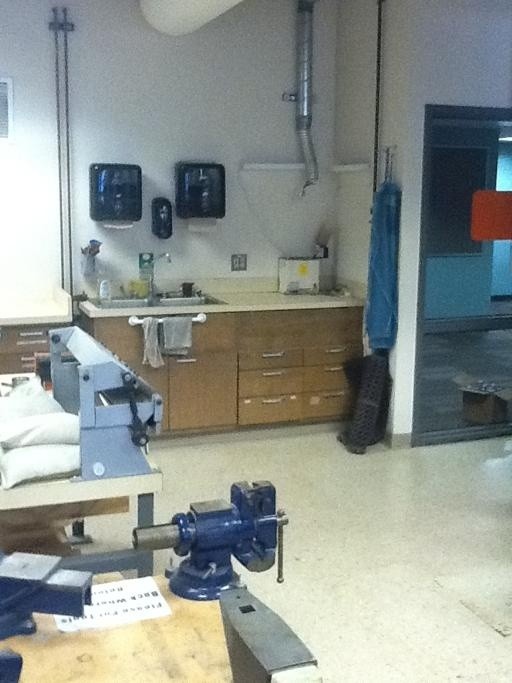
[2,316,73,374]
[81,306,366,439]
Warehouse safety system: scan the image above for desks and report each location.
[2,372,163,584]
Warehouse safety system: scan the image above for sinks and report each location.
[154,293,230,307]
[87,297,157,308]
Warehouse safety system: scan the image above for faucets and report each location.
[147,251,172,296]
